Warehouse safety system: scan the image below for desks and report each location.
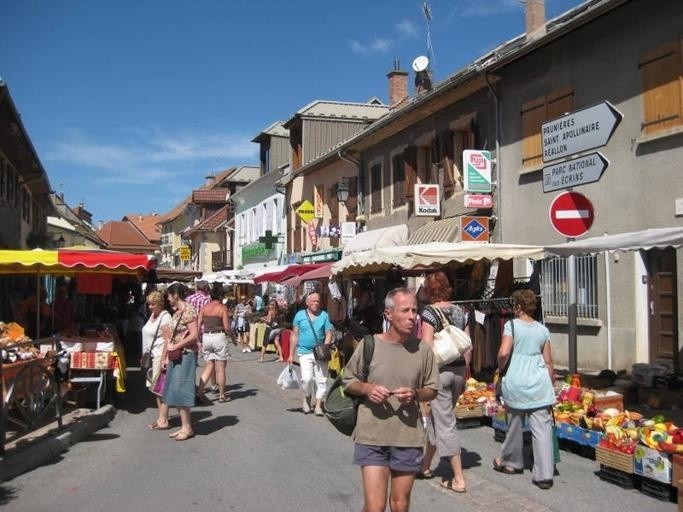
[0,354,63,455]
[69,353,116,411]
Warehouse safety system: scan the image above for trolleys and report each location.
[0,335,66,446]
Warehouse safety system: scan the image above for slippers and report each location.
[147,421,157,428]
[417,464,433,479]
[440,477,467,493]
[151,424,171,430]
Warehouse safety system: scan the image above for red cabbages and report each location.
[588,404,602,417]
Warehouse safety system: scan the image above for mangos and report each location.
[650,422,667,432]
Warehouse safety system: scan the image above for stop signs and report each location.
[546,191,594,238]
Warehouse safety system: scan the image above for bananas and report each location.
[569,413,580,425]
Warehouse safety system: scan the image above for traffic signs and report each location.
[540,152,607,191]
[540,102,623,160]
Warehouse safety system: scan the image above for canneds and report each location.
[566,374,581,388]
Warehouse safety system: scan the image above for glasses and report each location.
[241,298,246,300]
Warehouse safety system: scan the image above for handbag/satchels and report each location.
[423,305,474,370]
[139,316,163,373]
[319,331,373,434]
[494,319,516,397]
[303,309,332,362]
[166,314,185,361]
[548,411,561,464]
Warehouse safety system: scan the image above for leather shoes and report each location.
[170,428,182,438]
[175,430,194,441]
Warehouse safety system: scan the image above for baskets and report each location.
[594,445,633,474]
[455,402,484,420]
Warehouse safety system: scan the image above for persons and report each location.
[353,292,381,315]
[288,291,335,415]
[421,271,473,492]
[341,287,441,512]
[120,279,282,440]
[50,284,77,331]
[493,290,557,489]
[18,286,73,337]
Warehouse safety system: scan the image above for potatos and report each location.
[459,390,490,406]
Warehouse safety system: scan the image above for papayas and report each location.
[651,415,664,423]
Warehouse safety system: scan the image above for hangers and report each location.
[486,297,511,312]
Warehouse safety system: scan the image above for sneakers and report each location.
[195,391,205,401]
[492,456,515,474]
[532,477,553,490]
[314,407,323,416]
[220,394,232,402]
[302,397,313,414]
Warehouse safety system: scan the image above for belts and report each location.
[204,330,223,334]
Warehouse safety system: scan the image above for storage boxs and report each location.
[592,387,626,412]
[672,454,683,488]
[633,444,672,485]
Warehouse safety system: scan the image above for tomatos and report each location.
[600,439,636,454]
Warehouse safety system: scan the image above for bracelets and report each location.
[411,388,419,399]
